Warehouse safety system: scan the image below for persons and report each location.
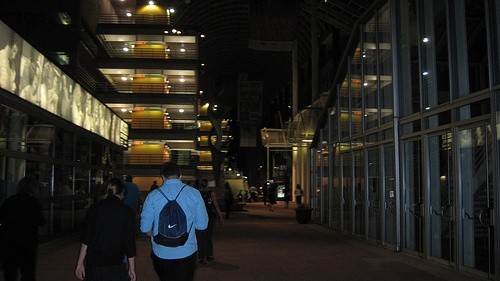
[237,189,258,201]
[140,162,209,280]
[0,176,48,281]
[74,177,136,281]
[123,174,142,213]
[295,183,304,209]
[223,182,235,218]
[194,178,224,263]
[150,180,158,191]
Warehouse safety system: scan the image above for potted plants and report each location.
[295,205,313,224]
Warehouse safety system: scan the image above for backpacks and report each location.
[153,184,193,247]
[201,191,214,222]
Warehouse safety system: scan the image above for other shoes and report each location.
[197,255,214,265]
